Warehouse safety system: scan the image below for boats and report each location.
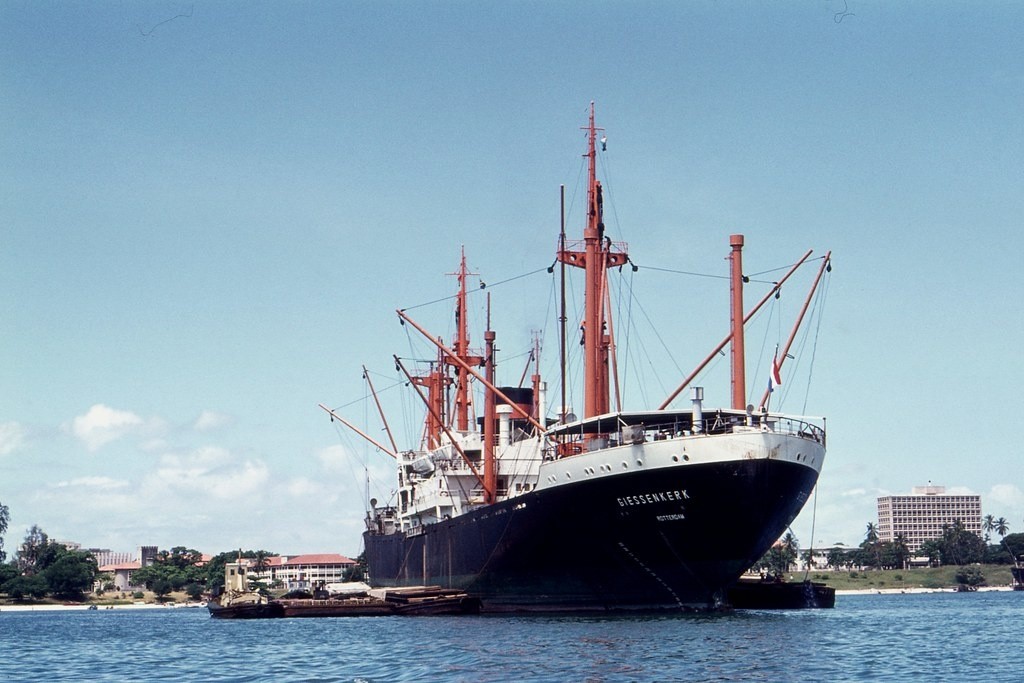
[205,567,476,616]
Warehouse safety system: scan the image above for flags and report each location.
[768,346,782,393]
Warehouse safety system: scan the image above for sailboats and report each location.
[317,100,846,616]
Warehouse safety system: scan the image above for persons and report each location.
[605,236,612,252]
